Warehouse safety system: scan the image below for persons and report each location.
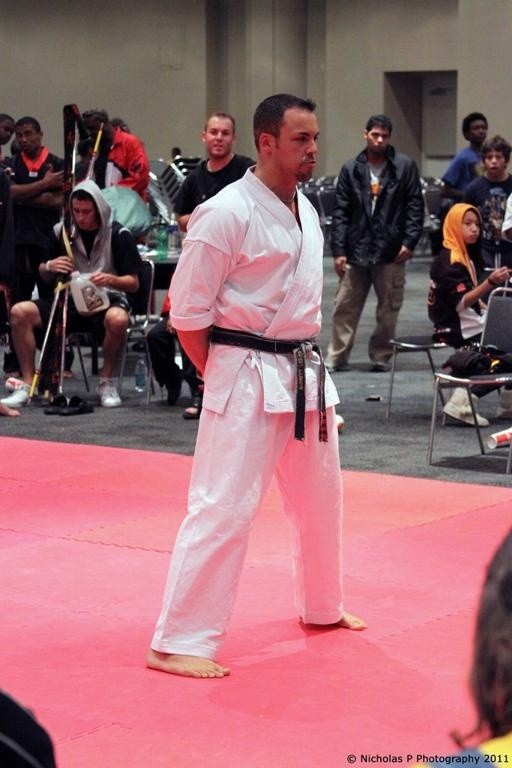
[144,94,368,679]
[321,113,425,373]
[1,108,149,410]
[173,112,257,424]
[148,290,205,420]
[412,528,512,768]
[427,112,511,427]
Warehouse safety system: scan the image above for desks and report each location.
[118,249,187,314]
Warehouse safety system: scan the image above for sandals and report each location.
[183,397,201,418]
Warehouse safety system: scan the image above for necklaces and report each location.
[281,187,296,203]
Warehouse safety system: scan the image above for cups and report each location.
[4,377,23,392]
[487,427,512,449]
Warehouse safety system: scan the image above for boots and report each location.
[443,386,489,427]
[496,387,512,419]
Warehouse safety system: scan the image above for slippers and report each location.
[44,395,93,415]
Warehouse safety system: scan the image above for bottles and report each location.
[167,213,179,250]
[156,225,168,260]
[134,356,148,393]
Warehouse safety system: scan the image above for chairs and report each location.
[426,287,512,473]
[302,175,444,260]
[63,257,160,407]
[386,333,461,419]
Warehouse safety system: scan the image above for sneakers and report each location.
[96,375,121,408]
[0,390,34,408]
[324,362,350,373]
[165,368,184,404]
[370,362,391,372]
